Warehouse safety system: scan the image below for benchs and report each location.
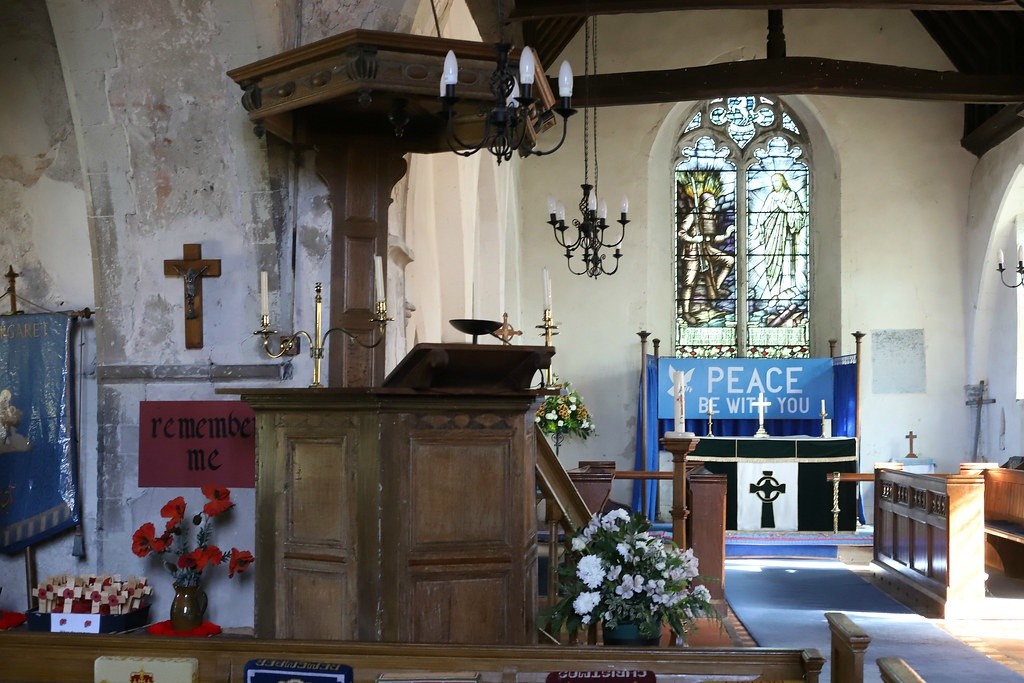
[960,468,1024,577]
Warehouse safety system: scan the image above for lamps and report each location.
[997,244,1024,288]
[439,1,578,167]
[547,15,631,281]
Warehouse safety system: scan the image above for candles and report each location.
[261,272,269,316]
[374,255,386,302]
[759,393,763,425]
[821,399,825,415]
[543,266,552,310]
[672,369,685,433]
[708,397,713,413]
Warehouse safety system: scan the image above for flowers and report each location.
[537,380,598,441]
[131,482,254,589]
[534,505,724,649]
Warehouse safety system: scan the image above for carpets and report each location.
[723,560,1024,683]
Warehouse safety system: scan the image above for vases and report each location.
[170,585,208,631]
[601,612,660,647]
